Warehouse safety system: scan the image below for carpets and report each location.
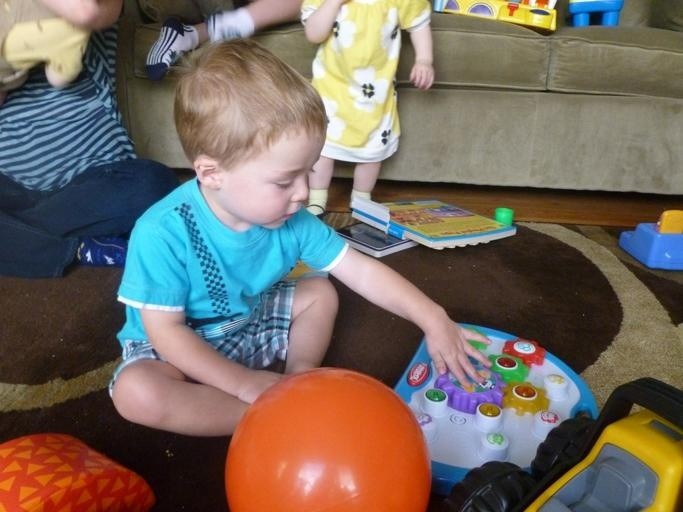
[0,210,682,512]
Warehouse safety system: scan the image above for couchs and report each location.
[113,0,681,195]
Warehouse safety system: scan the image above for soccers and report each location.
[224,368,433,508]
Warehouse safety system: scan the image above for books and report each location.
[334,222,419,259]
[348,198,517,250]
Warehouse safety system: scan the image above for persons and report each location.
[144,1,304,83]
[299,0,436,220]
[106,34,495,439]
[0,1,182,280]
